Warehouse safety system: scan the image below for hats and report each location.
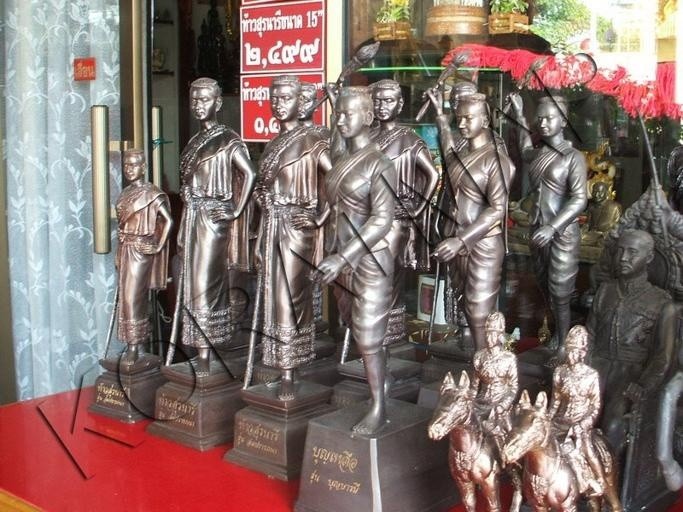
[485,312,507,333]
[565,325,589,350]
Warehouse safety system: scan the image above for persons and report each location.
[548,325,623,496]
[339,79,438,368]
[431,92,511,351]
[103,149,173,365]
[469,311,522,512]
[163,77,255,378]
[587,229,677,505]
[298,41,383,146]
[310,86,398,436]
[243,75,333,402]
[511,96,587,368]
